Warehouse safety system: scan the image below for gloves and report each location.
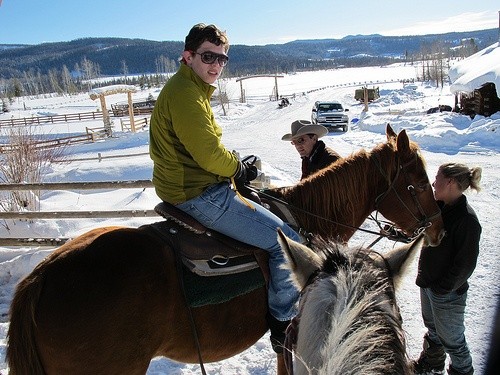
[234,161,257,181]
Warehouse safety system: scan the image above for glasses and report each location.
[291,138,311,145]
[188,50,229,67]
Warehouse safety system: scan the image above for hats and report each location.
[282,120,328,141]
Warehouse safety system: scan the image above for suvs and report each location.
[311,100,350,132]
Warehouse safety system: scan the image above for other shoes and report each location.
[407,357,445,375]
[270,336,285,355]
[447,365,474,375]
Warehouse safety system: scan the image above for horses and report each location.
[276,226,427,375]
[7,125,447,375]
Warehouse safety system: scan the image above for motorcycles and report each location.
[278,97,293,108]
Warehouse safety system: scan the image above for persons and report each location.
[282,120,341,182]
[149,23,303,355]
[409,162,482,375]
